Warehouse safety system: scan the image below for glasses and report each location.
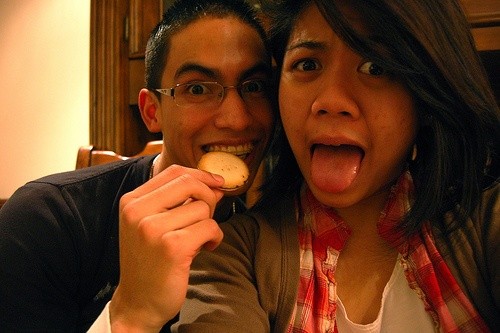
[152,79,280,110]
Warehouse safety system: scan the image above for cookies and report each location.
[196,152,250,191]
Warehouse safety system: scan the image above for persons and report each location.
[167,1,500,332]
[1,1,285,333]
